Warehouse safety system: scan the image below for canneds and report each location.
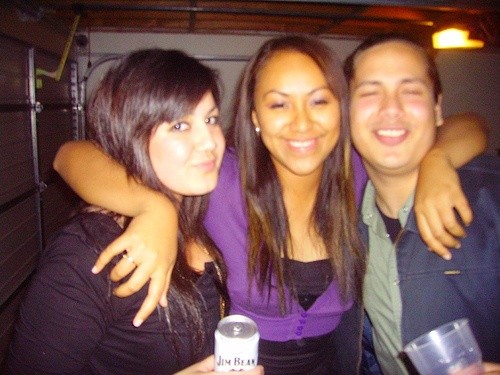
[213,314,260,372]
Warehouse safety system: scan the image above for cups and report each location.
[405,318,483,375]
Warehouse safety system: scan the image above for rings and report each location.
[122,253,138,269]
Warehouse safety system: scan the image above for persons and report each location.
[0,47,265,373]
[52,34,488,374]
[321,34,500,374]
